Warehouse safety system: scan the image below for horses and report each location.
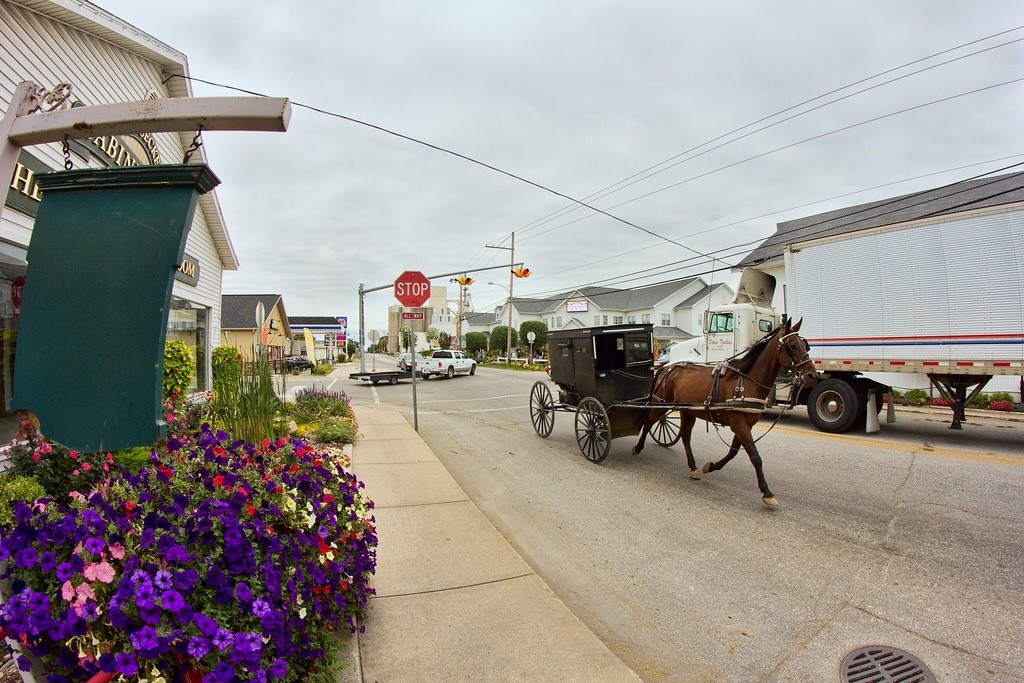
[631,315,820,507]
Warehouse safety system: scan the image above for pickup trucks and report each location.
[416,350,477,379]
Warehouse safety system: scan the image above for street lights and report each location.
[488,282,512,365]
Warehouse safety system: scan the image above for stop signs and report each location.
[394,271,431,308]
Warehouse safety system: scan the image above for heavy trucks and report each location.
[654,202,1024,442]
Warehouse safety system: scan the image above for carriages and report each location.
[531,310,822,507]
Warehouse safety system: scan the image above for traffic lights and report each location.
[511,268,532,278]
[455,277,476,285]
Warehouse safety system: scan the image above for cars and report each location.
[396,353,409,367]
[401,353,425,372]
